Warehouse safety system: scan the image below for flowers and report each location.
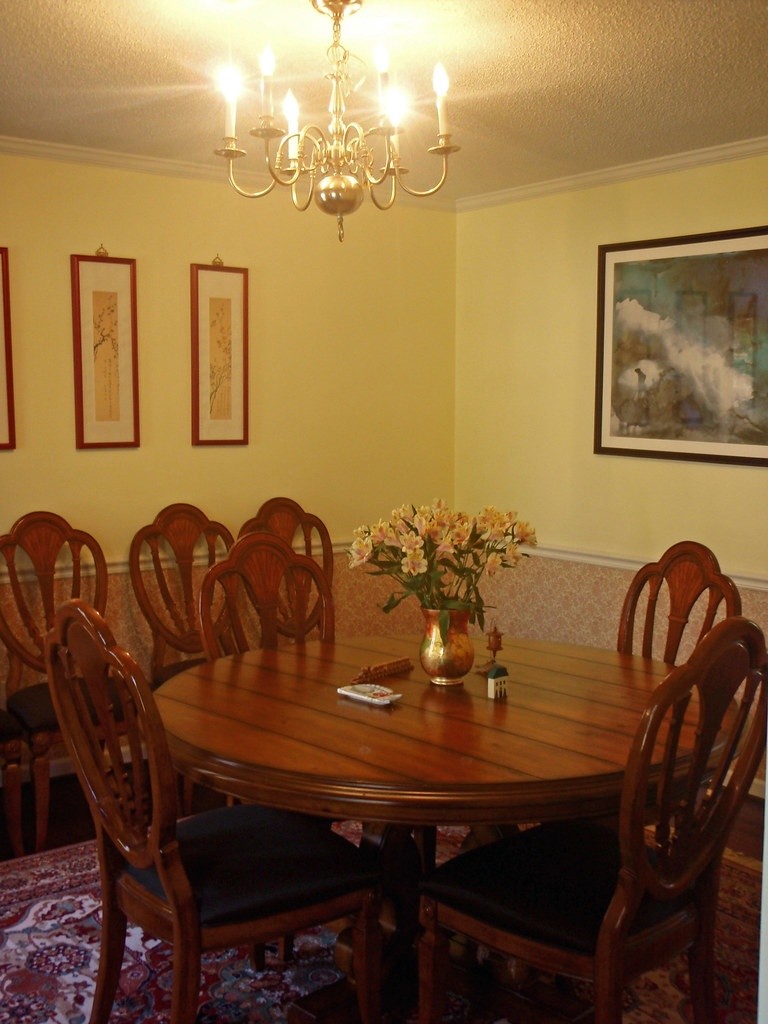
[348,497,537,646]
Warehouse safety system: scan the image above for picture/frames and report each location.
[593,225,768,468]
[191,263,248,446]
[68,254,141,449]
[0,246,16,450]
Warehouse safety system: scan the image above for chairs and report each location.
[40,600,384,1024]
[127,502,235,817]
[238,497,333,636]
[618,541,742,665]
[0,511,147,854]
[416,616,768,1024]
[192,530,337,663]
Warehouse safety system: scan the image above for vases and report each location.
[419,604,475,685]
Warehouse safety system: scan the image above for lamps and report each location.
[209,0,460,242]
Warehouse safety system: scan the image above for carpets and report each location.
[0,823,761,1024]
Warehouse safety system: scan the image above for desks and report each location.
[137,635,749,1023]
